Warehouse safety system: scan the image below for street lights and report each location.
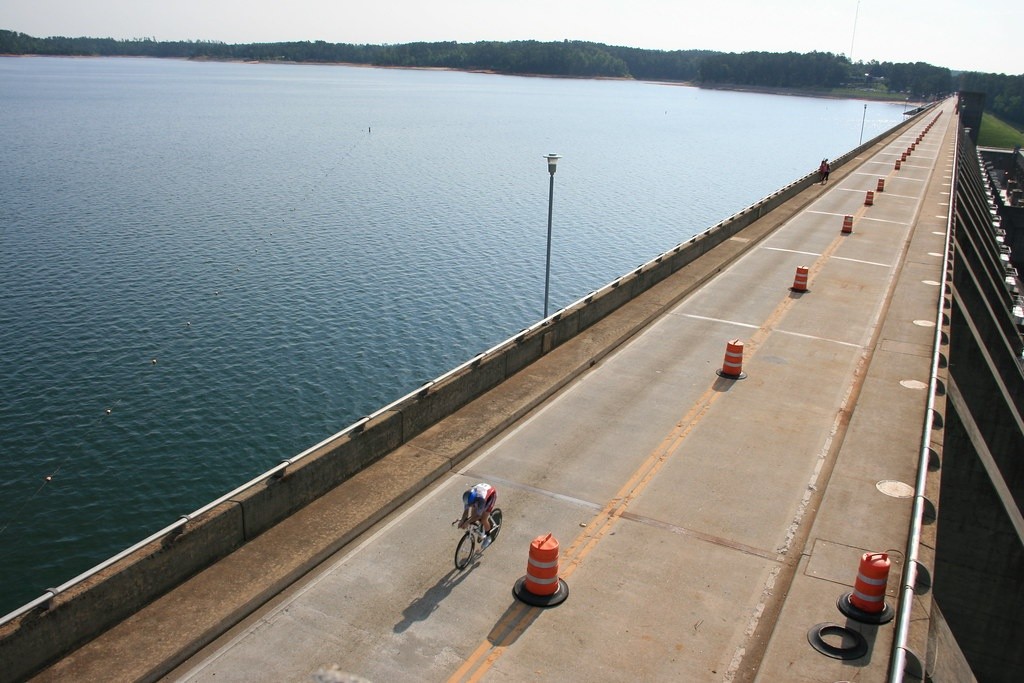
[542,151,562,320]
[902,97,909,122]
[859,103,869,145]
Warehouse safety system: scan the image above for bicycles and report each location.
[454,506,504,573]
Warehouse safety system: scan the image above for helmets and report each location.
[463,489,477,505]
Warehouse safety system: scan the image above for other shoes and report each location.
[481,537,491,549]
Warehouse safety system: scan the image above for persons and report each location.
[456,483,497,549]
[820,159,831,182]
[1014,144,1021,153]
